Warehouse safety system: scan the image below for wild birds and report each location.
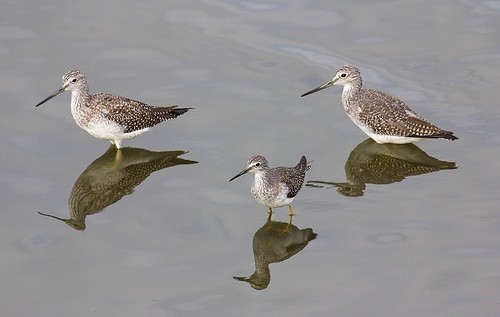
[231,214,317,290]
[305,137,460,196]
[301,65,458,144]
[229,154,313,215]
[38,144,198,230]
[35,70,193,149]
